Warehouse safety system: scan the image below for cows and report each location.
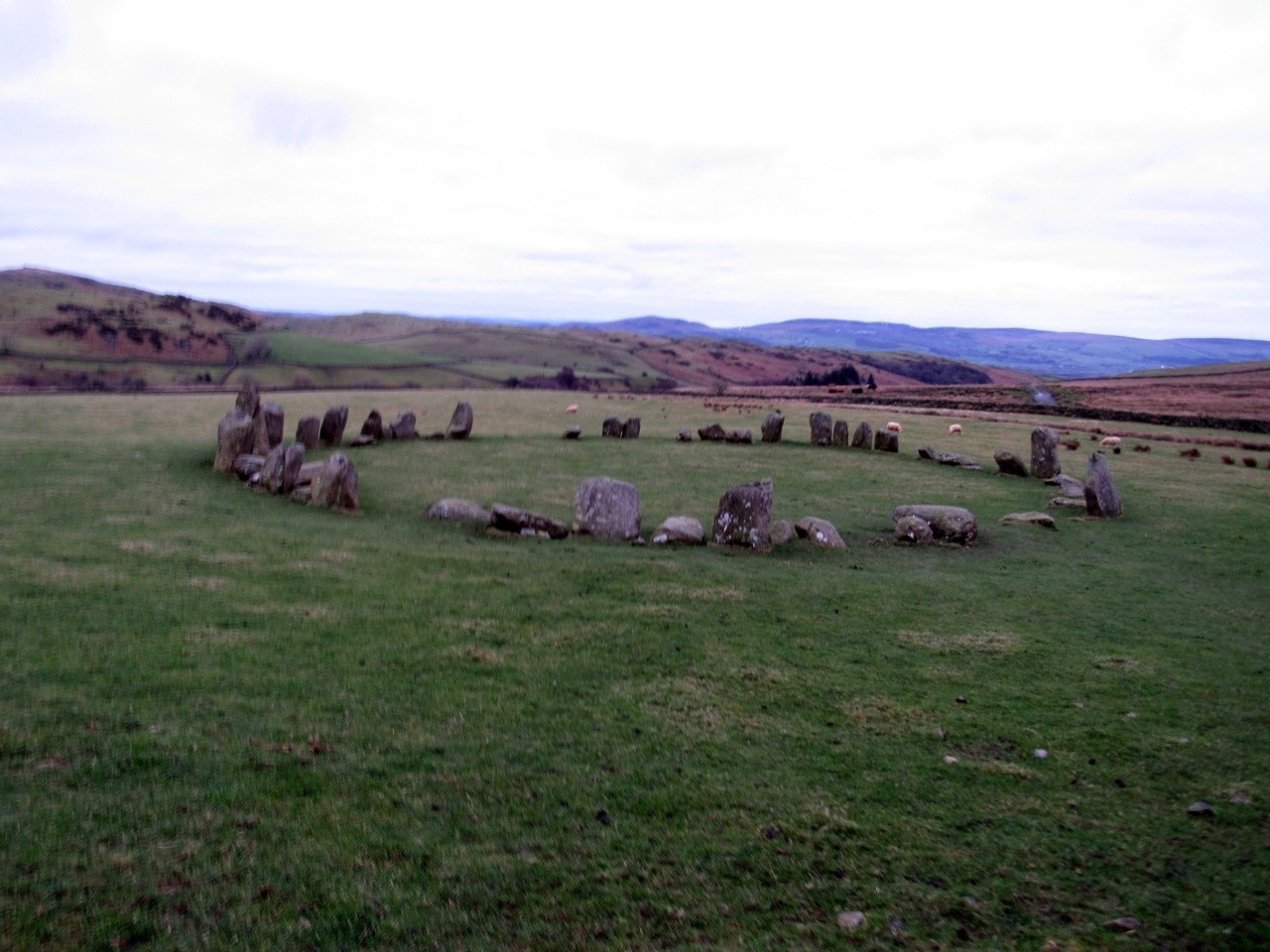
[949,423,961,435]
[1099,435,1121,448]
[566,403,579,413]
[887,422,901,433]
